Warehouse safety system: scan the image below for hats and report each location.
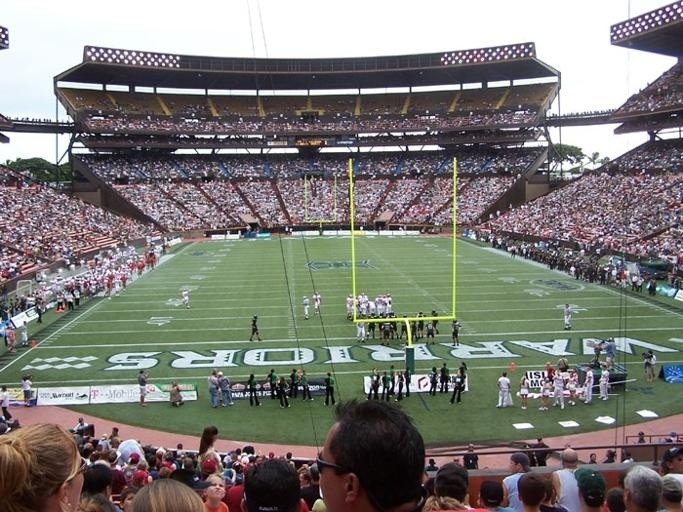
[129,453,140,465]
[661,475,682,494]
[664,447,682,462]
[287,451,292,459]
[268,451,274,458]
[169,468,211,491]
[511,453,532,472]
[574,466,605,498]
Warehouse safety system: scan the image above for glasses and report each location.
[316,451,356,475]
[47,455,88,498]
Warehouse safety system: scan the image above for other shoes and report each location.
[538,393,612,410]
[521,406,527,410]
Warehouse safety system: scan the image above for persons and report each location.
[300,295,308,318]
[345,291,439,346]
[563,303,572,330]
[0,164,171,348]
[450,319,462,347]
[312,291,320,316]
[182,288,189,309]
[248,315,262,341]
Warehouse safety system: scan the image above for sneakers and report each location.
[210,398,339,408]
[292,313,464,350]
